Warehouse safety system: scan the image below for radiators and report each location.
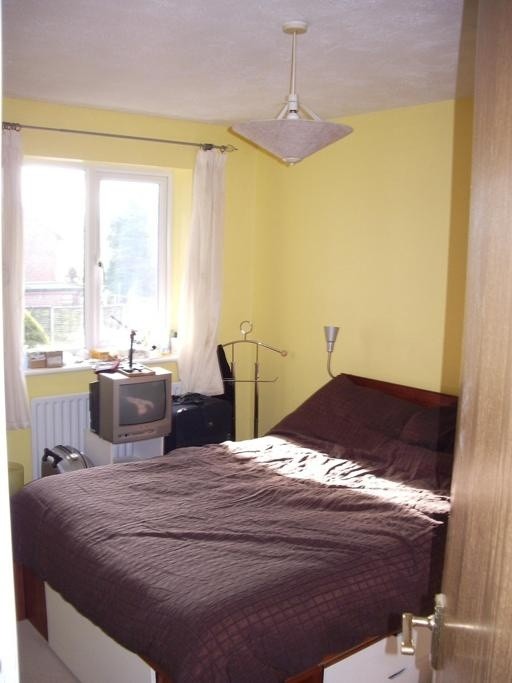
[28,383,180,476]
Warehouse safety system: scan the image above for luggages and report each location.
[168,392,234,449]
[41,445,94,475]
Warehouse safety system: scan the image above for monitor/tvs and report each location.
[88,366,172,445]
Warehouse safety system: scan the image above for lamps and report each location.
[223,16,361,167]
[322,325,341,380]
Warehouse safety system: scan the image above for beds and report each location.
[19,372,459,680]
